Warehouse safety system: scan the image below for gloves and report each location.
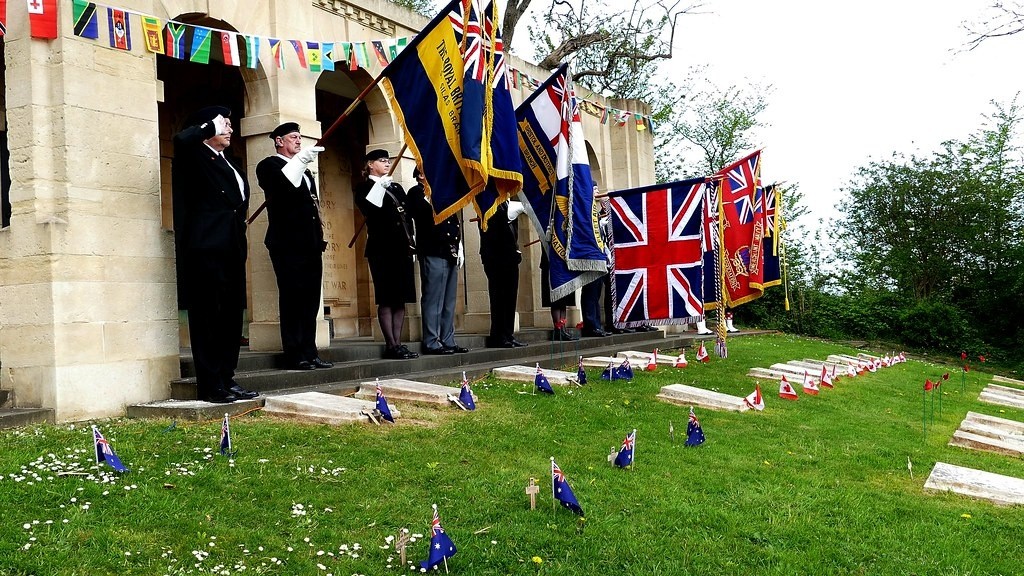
[456,241,464,269]
[507,201,527,221]
[281,146,325,188]
[366,174,393,208]
[213,114,231,136]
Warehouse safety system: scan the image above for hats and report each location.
[364,149,389,161]
[269,122,300,140]
[198,105,230,123]
[412,165,421,178]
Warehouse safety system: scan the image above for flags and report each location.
[534,339,908,469]
[607,149,785,327]
[376,0,523,229]
[552,463,584,517]
[220,419,233,456]
[505,64,655,134]
[27,0,260,70]
[419,510,457,571]
[93,429,132,473]
[460,377,475,410]
[375,384,395,423]
[268,33,415,74]
[512,61,611,301]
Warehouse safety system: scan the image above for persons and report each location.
[406,165,468,355]
[696,315,714,335]
[725,306,741,333]
[256,121,335,369]
[582,181,658,337]
[352,150,419,359]
[479,201,528,348]
[172,106,260,403]
[538,245,578,341]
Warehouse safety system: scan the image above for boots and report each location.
[725,319,740,333]
[697,319,714,335]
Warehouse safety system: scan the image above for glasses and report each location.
[380,158,391,163]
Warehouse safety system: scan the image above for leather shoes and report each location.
[499,340,514,348]
[422,346,455,355]
[296,360,316,370]
[445,345,468,353]
[197,386,237,403]
[510,338,529,347]
[382,345,410,359]
[311,357,332,368]
[224,381,259,398]
[400,344,419,359]
[582,324,660,337]
[555,329,579,341]
